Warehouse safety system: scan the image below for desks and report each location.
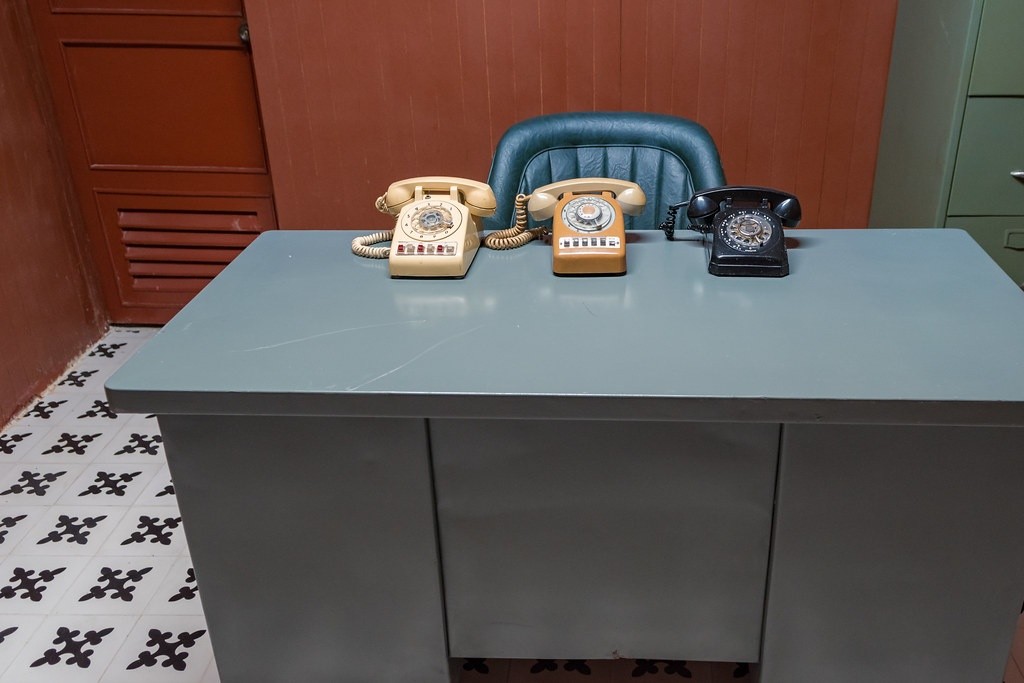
[104,228,1024,683]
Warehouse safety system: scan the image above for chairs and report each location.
[483,110,727,231]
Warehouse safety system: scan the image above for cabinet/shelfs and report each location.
[868,0,1024,289]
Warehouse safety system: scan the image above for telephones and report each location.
[686,185,804,277]
[381,175,497,277]
[527,176,648,276]
